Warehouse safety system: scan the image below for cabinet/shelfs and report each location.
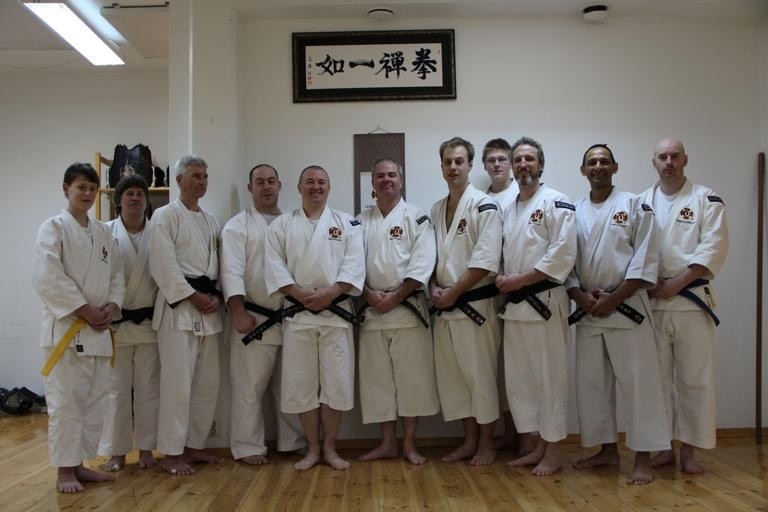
[94,150,169,221]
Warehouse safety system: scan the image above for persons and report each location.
[479,136,539,457]
[102,170,158,474]
[354,157,439,468]
[31,163,127,494]
[219,164,311,470]
[146,151,225,476]
[634,133,731,478]
[500,137,580,479]
[264,162,367,473]
[564,141,673,486]
[429,136,506,466]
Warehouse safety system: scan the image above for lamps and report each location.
[21,0,131,75]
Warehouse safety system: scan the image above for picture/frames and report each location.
[291,28,457,104]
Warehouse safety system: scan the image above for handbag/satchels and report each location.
[109,144,152,189]
